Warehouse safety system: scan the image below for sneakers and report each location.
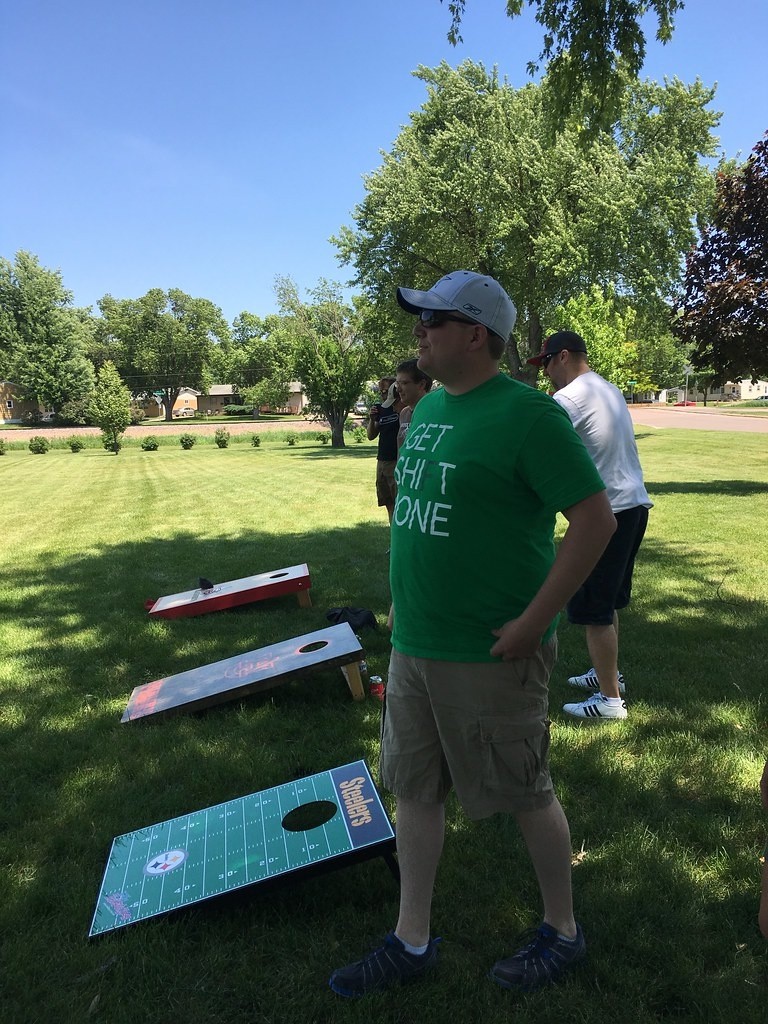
[493,921,588,993]
[563,691,627,719]
[568,667,625,695]
[330,930,444,998]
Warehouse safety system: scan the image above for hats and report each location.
[396,272,518,344]
[381,380,400,408]
[527,331,587,366]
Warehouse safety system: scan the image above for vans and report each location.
[753,394,768,403]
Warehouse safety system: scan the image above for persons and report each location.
[396,359,434,464]
[324,268,621,991]
[528,330,652,720]
[387,380,407,414]
[367,377,408,527]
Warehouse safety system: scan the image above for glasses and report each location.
[541,353,559,369]
[420,309,493,337]
[379,389,390,394]
[395,381,421,389]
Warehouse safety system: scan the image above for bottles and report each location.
[355,634,362,645]
[359,658,367,675]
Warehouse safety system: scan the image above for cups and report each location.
[370,403,383,422]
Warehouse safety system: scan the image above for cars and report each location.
[354,402,369,415]
[39,411,56,423]
[173,407,195,417]
[673,400,696,407]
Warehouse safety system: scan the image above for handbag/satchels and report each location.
[327,605,376,637]
